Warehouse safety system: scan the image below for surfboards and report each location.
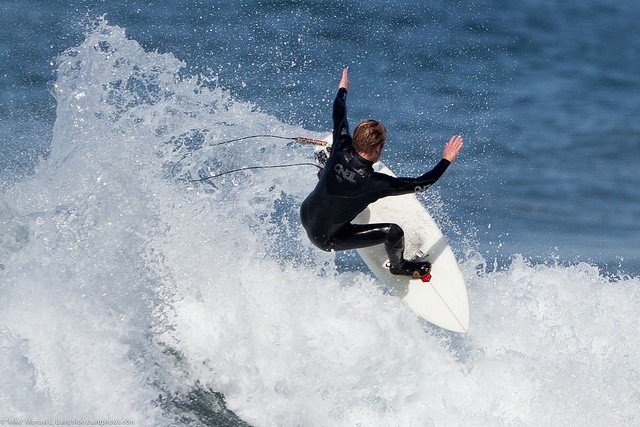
[314,134,469,333]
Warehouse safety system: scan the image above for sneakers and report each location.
[390,258,431,278]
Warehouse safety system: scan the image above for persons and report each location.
[300,67,463,277]
